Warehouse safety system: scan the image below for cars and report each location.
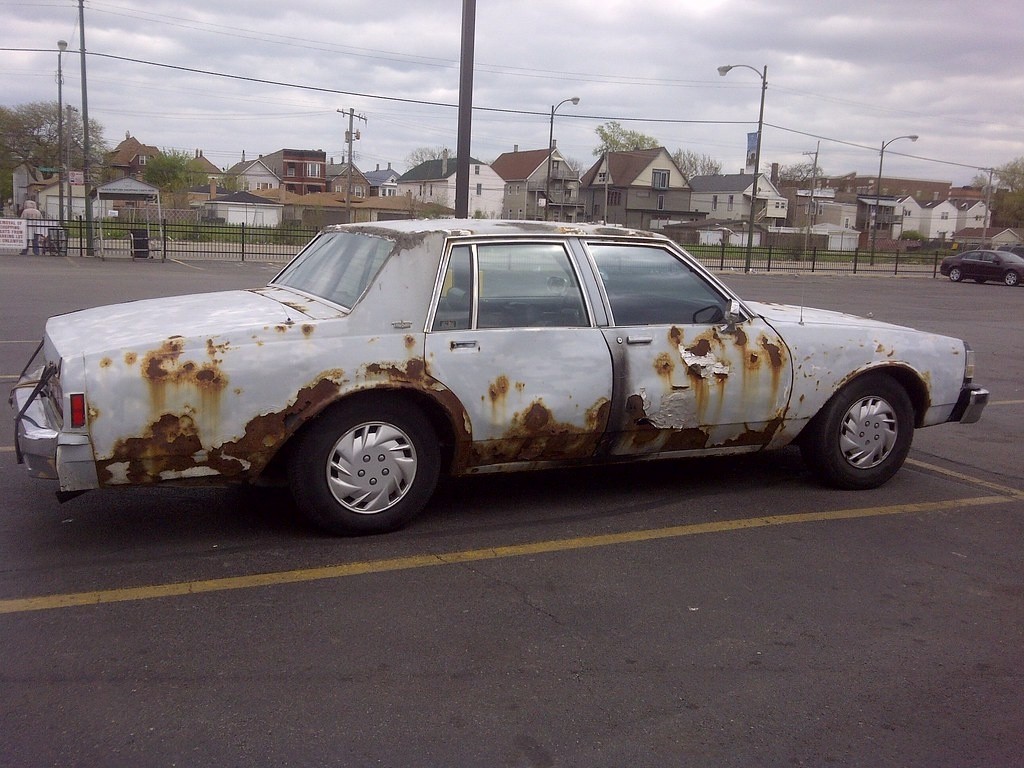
[939,250,1024,287]
[8,219,989,538]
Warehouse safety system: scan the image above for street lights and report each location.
[718,64,768,273]
[870,136,918,267]
[545,98,580,221]
[57,40,67,225]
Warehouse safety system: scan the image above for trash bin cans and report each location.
[131,229,149,258]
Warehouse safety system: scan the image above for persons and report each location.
[19,200,43,255]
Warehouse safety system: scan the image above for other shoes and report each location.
[19,252,25,255]
